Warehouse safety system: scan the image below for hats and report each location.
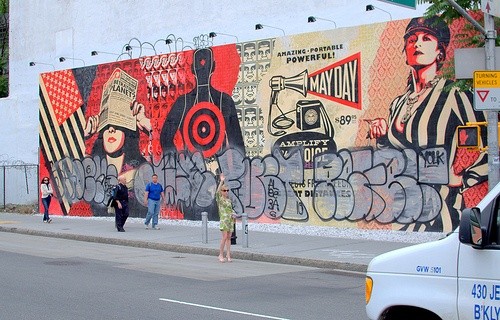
[403,15,451,50]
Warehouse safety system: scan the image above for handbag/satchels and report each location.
[107,196,115,208]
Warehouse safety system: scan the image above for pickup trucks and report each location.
[364,181,500,320]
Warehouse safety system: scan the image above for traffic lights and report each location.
[457,125,482,150]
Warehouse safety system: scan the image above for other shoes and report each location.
[146,226,150,230]
[47,218,52,224]
[218,255,224,263]
[153,224,160,230]
[225,256,232,263]
[43,220,47,223]
[115,226,125,232]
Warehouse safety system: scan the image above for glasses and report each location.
[44,180,49,182]
[222,189,229,193]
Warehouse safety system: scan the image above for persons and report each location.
[144,173,166,230]
[111,176,129,232]
[216,172,233,263]
[40,177,52,223]
